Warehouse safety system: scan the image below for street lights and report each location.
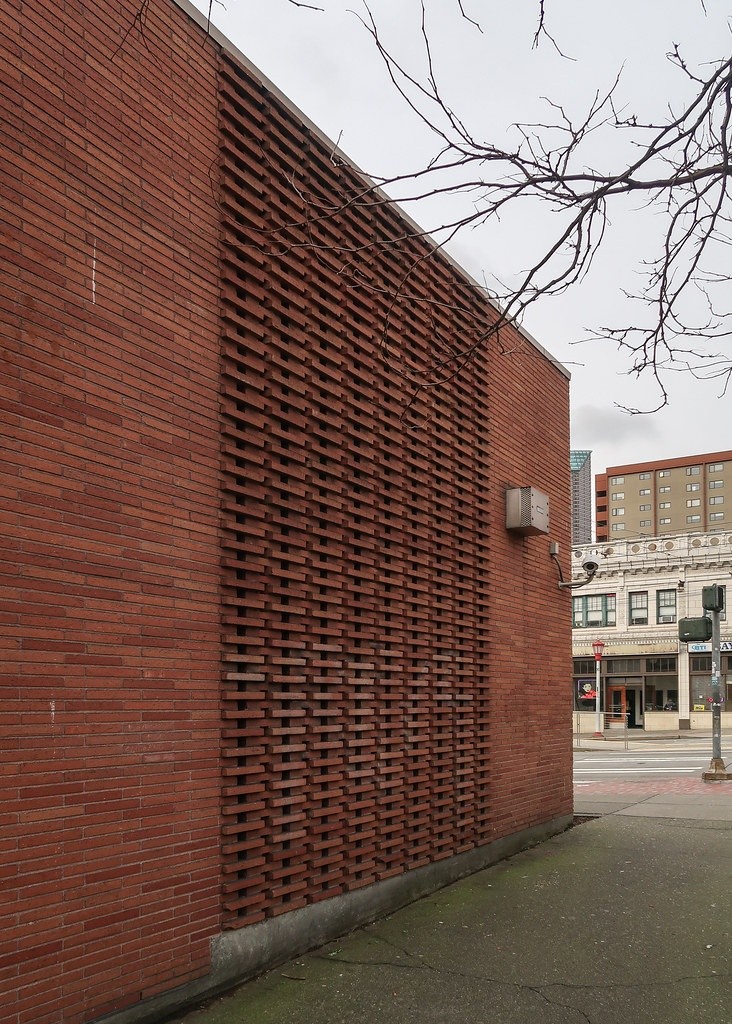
[592,638,605,738]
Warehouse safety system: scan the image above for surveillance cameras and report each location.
[581,555,600,572]
